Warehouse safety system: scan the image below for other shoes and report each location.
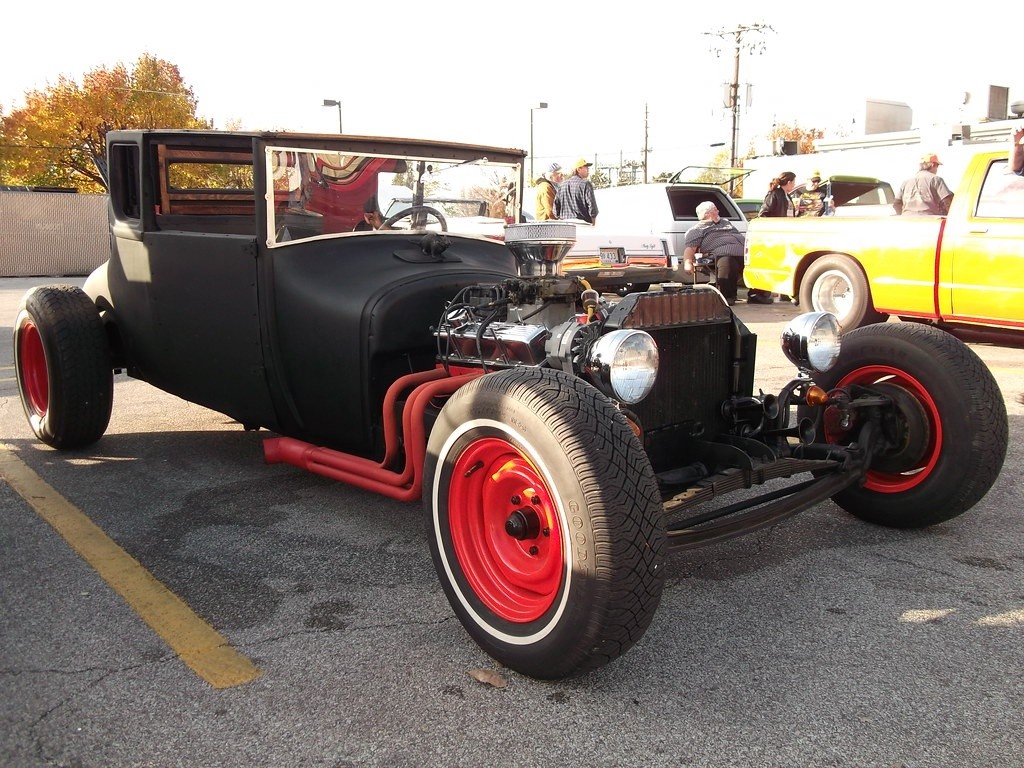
[747,292,773,304]
[726,296,735,306]
[780,295,791,301]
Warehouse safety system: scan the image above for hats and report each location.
[806,170,821,177]
[575,159,593,170]
[547,162,562,172]
[920,153,943,165]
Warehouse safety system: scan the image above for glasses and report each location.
[810,179,821,184]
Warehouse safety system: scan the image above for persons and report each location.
[352,193,391,232]
[1010,126,1024,175]
[796,171,835,218]
[683,201,774,306]
[758,171,797,217]
[535,159,599,226]
[892,154,954,215]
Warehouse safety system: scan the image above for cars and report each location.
[14,129,1010,681]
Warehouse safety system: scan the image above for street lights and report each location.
[324,100,342,134]
[531,102,547,180]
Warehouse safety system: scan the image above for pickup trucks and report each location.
[743,152,1024,342]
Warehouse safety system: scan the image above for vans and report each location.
[521,166,755,286]
